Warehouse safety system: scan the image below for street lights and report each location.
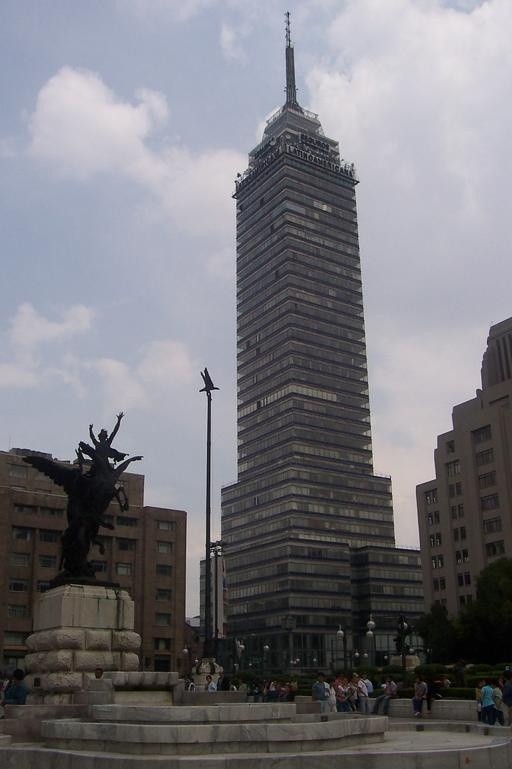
[205,538,226,640]
[350,646,369,669]
[336,622,350,672]
[289,653,303,666]
[197,363,220,654]
[367,611,379,669]
[182,646,193,674]
[258,638,272,679]
[397,612,415,690]
[312,655,319,665]
[384,652,392,660]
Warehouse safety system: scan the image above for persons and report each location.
[95,669,103,679]
[89,411,126,475]
[190,669,297,703]
[312,668,510,726]
[2,670,27,704]
